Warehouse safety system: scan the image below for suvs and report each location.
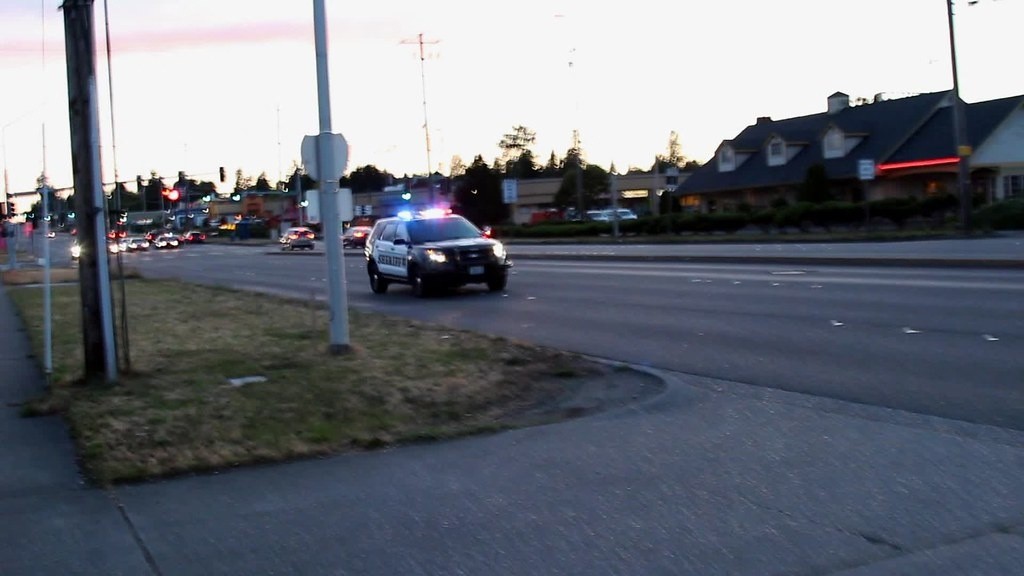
[279,227,316,251]
[363,206,514,299]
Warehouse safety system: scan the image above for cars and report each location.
[530,206,639,223]
[343,226,374,249]
[68,229,206,262]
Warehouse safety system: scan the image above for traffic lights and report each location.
[7,201,16,218]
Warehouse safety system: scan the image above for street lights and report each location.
[945,0,980,235]
